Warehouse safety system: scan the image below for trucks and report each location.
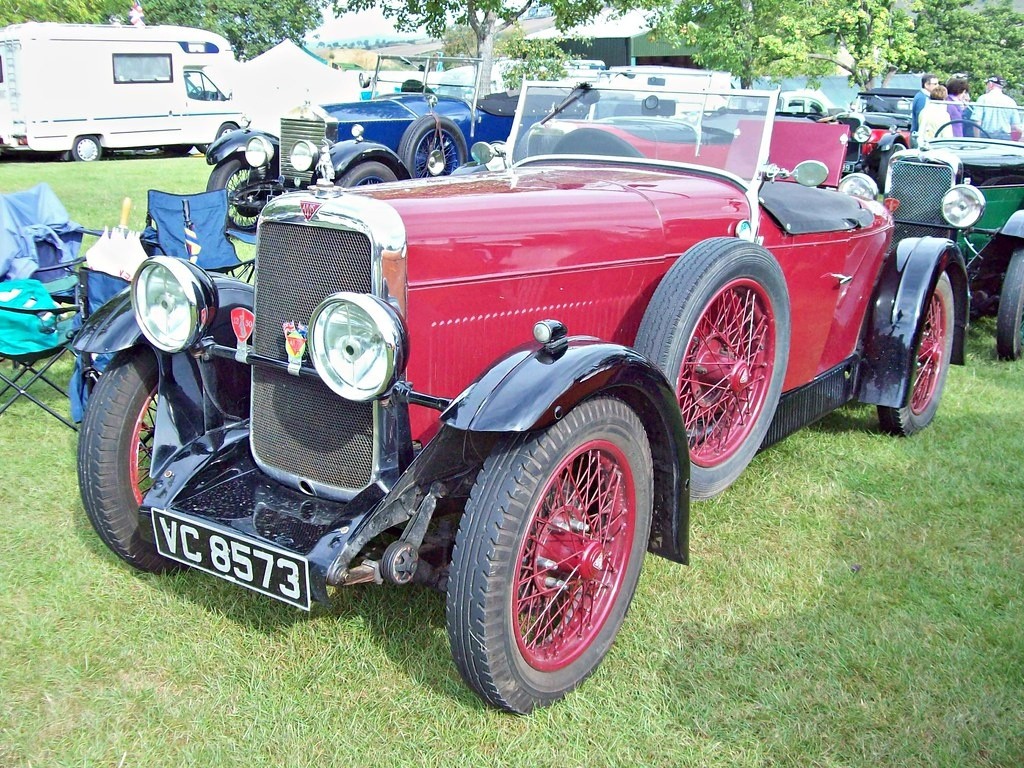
[0,24,252,165]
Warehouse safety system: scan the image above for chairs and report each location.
[724,118,849,190]
[144,189,257,285]
[1,181,157,449]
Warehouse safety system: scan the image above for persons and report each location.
[910,72,1022,148]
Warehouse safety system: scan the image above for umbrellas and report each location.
[85,197,148,283]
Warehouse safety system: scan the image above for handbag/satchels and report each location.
[0,279,73,355]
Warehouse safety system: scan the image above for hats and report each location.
[984,75,1007,88]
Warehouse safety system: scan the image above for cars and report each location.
[73,77,974,720]
[203,52,1023,360]
